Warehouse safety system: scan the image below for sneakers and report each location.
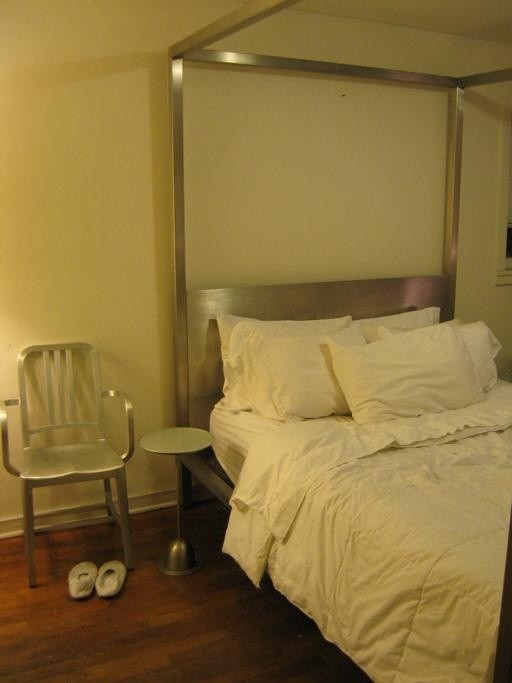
[68,562,98,599]
[96,559,127,597]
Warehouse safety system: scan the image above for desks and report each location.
[134,425,218,580]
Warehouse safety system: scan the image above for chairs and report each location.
[0,337,140,589]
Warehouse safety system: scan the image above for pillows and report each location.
[352,301,442,344]
[226,313,503,427]
[212,306,356,414]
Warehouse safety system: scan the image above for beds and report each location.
[164,0,512,681]
